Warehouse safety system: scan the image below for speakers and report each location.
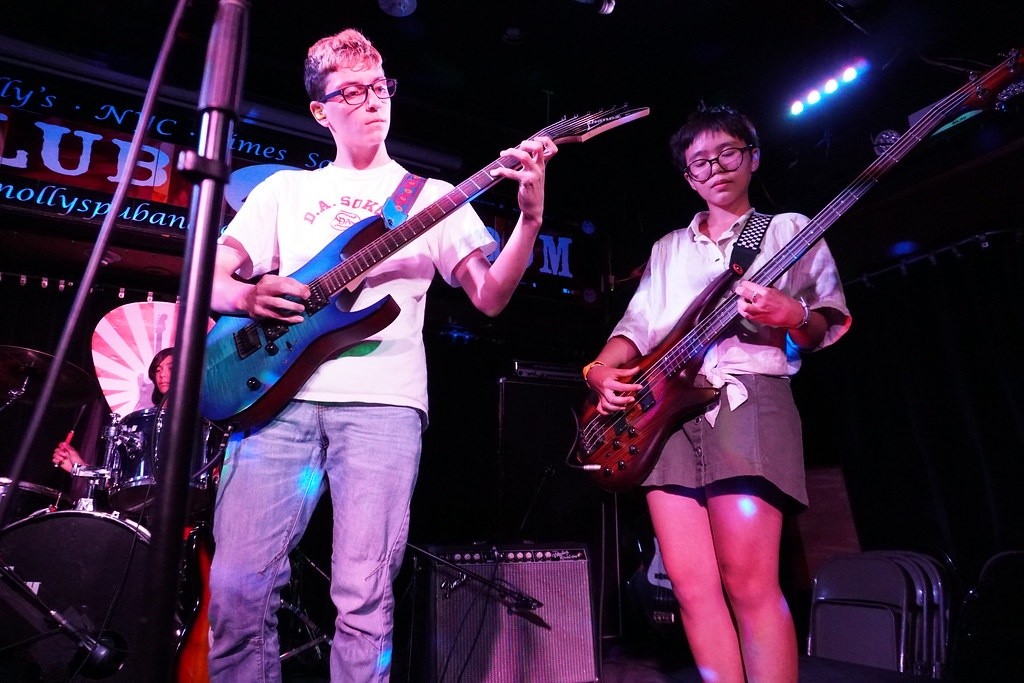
[494,374,613,650]
[430,560,595,683]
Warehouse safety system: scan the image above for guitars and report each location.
[625,534,680,626]
[576,46,1023,492]
[200,104,652,437]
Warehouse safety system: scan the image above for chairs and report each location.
[808,550,956,679]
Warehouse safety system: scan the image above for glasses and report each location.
[316,77,398,105]
[682,143,754,182]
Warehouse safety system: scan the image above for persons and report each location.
[579,107,852,683]
[209,30,558,683]
[52,347,175,479]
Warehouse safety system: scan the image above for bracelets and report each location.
[582,361,609,390]
[796,296,810,331]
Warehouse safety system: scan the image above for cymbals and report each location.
[0,344,101,409]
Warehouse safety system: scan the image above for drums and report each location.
[102,409,215,515]
[67,461,108,513]
[0,508,151,683]
[0,477,63,529]
